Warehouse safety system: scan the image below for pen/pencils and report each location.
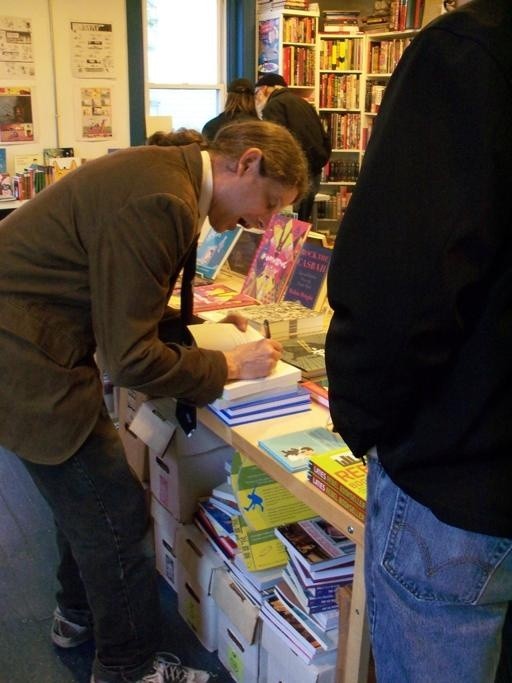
[264,319,270,339]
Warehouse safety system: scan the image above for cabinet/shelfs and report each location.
[313,32,371,242]
[101,285,379,681]
[358,26,426,174]
[249,0,320,122]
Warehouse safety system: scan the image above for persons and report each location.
[0,121,310,682]
[325,2,512,681]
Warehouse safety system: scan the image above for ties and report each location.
[176,243,197,439]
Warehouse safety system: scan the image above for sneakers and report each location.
[90,651,213,683]
[50,603,92,648]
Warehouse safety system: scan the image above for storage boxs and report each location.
[113,383,375,682]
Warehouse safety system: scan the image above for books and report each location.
[0,147,80,204]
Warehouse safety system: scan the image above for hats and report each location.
[228,78,255,95]
[255,74,287,87]
